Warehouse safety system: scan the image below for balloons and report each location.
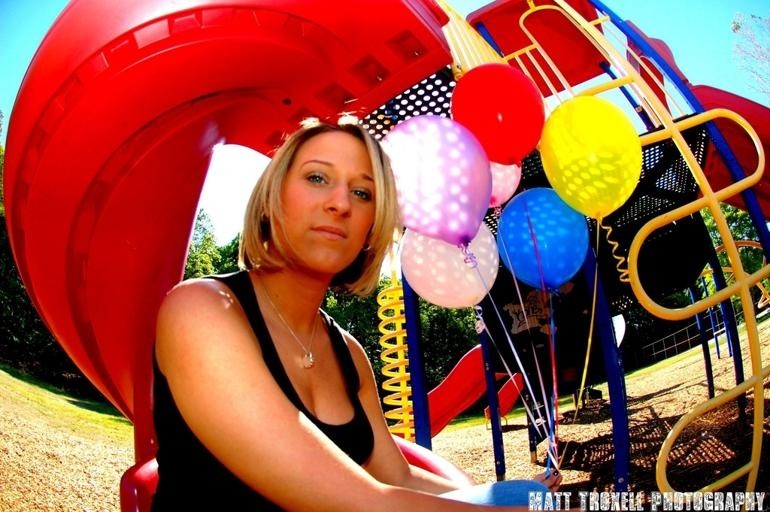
[496,186,591,294]
[399,223,503,312]
[378,115,492,250]
[538,95,644,223]
[485,159,522,211]
[451,62,547,165]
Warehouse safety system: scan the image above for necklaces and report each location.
[257,272,328,368]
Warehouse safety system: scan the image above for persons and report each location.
[145,123,565,511]
